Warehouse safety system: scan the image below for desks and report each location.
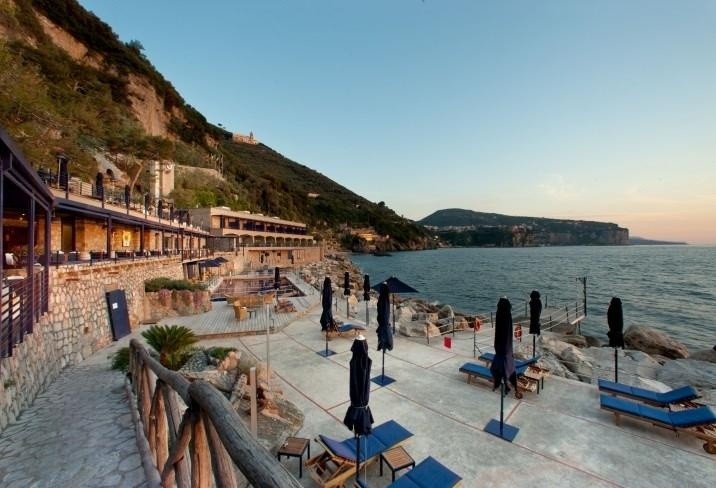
[527,374,544,394]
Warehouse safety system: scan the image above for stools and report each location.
[278,437,310,478]
[380,446,415,483]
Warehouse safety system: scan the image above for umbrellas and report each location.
[275,267,280,301]
[529,289,542,358]
[343,271,351,319]
[363,273,371,327]
[606,296,625,383]
[377,282,394,379]
[319,276,333,352]
[199,257,229,279]
[57,153,193,225]
[344,334,374,485]
[489,295,516,425]
[371,275,418,333]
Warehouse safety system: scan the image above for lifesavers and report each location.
[514,327,522,338]
[474,319,480,331]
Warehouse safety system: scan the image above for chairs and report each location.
[478,352,548,375]
[388,455,462,488]
[459,362,537,398]
[598,377,702,405]
[600,394,716,454]
[224,291,275,321]
[304,419,415,488]
